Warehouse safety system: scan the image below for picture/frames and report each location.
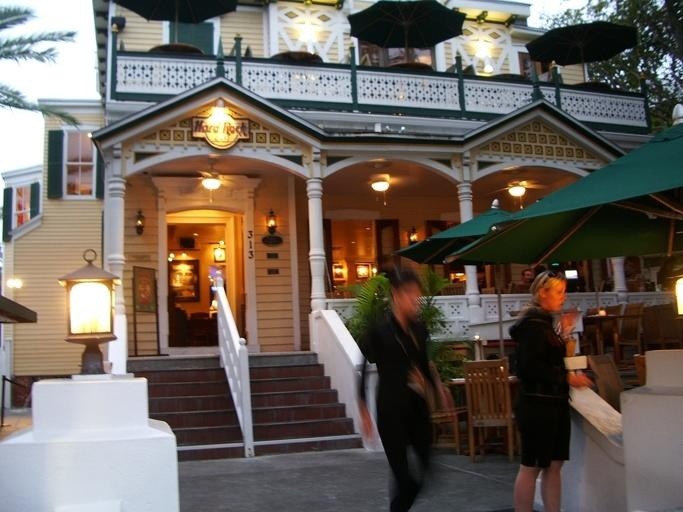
[166,257,202,304]
[213,246,226,263]
[353,261,371,281]
[331,263,346,284]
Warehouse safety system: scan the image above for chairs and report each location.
[463,357,518,460]
[575,302,679,371]
[421,360,484,453]
[588,353,647,412]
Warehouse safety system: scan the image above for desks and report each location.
[143,43,613,92]
[449,376,521,450]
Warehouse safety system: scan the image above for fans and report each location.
[190,168,241,194]
[485,178,550,196]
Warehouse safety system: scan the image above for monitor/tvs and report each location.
[565,268,578,281]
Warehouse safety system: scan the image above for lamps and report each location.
[55,247,124,376]
[203,99,236,131]
[133,207,147,236]
[664,272,682,320]
[504,180,528,198]
[200,178,221,189]
[262,207,282,238]
[367,172,396,209]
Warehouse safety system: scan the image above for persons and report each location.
[354,268,459,511]
[507,271,595,511]
[517,271,532,286]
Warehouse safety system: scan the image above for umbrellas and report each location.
[523,21,638,83]
[392,197,521,362]
[345,0,467,63]
[114,1,240,42]
[442,104,683,266]
